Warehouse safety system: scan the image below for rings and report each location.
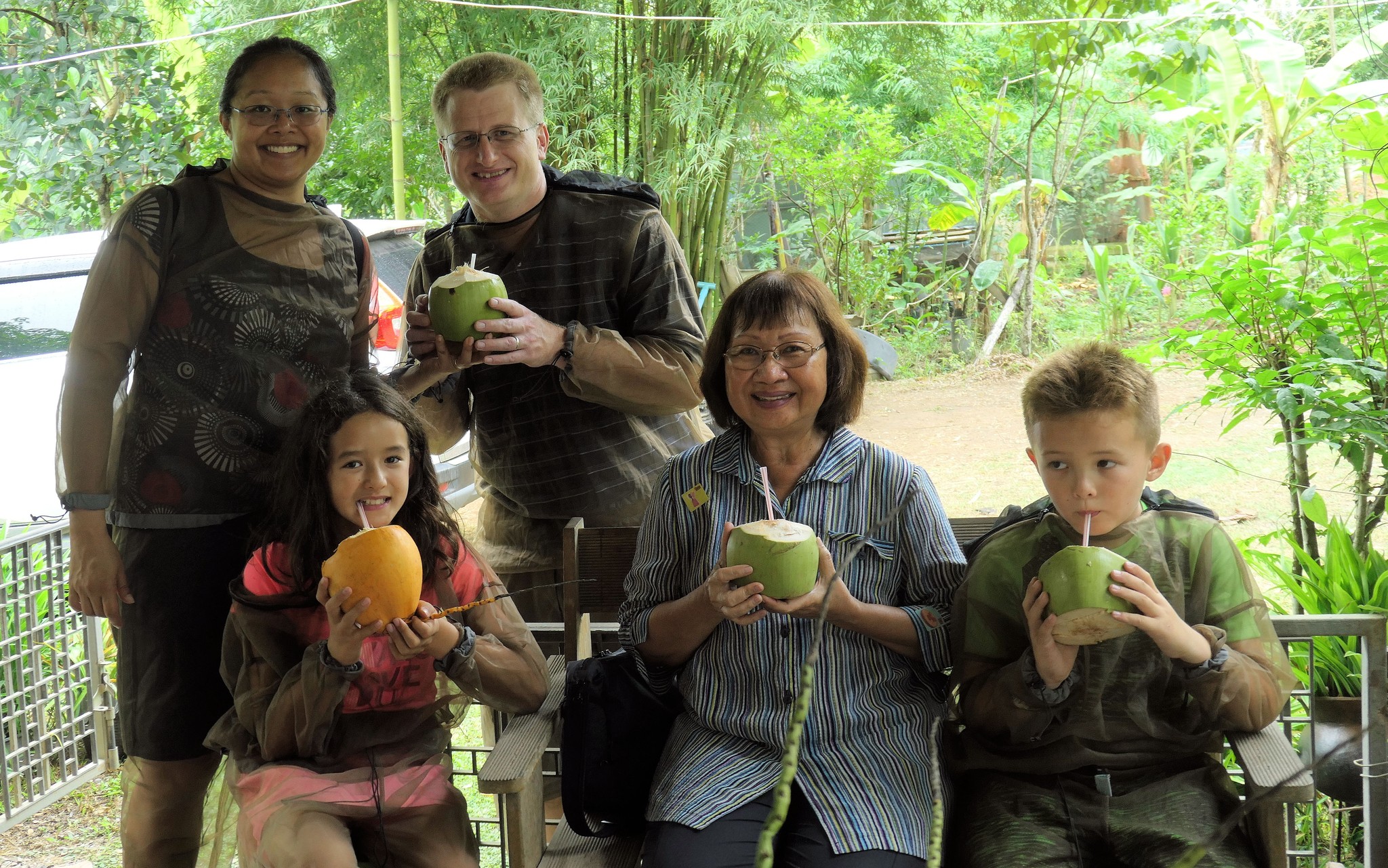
[512,336,519,351]
[454,356,464,369]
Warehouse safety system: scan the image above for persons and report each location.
[405,57,727,739]
[615,264,973,867]
[57,35,371,867]
[219,372,552,867]
[951,339,1291,868]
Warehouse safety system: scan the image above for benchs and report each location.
[478,506,1316,868]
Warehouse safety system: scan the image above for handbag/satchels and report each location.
[563,648,678,847]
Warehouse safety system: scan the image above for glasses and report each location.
[723,341,827,371]
[226,103,329,127]
[440,122,540,153]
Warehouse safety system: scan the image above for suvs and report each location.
[2,201,482,536]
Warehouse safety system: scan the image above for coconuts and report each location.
[321,525,422,635]
[1037,545,1143,645]
[726,519,819,599]
[428,262,508,342]
